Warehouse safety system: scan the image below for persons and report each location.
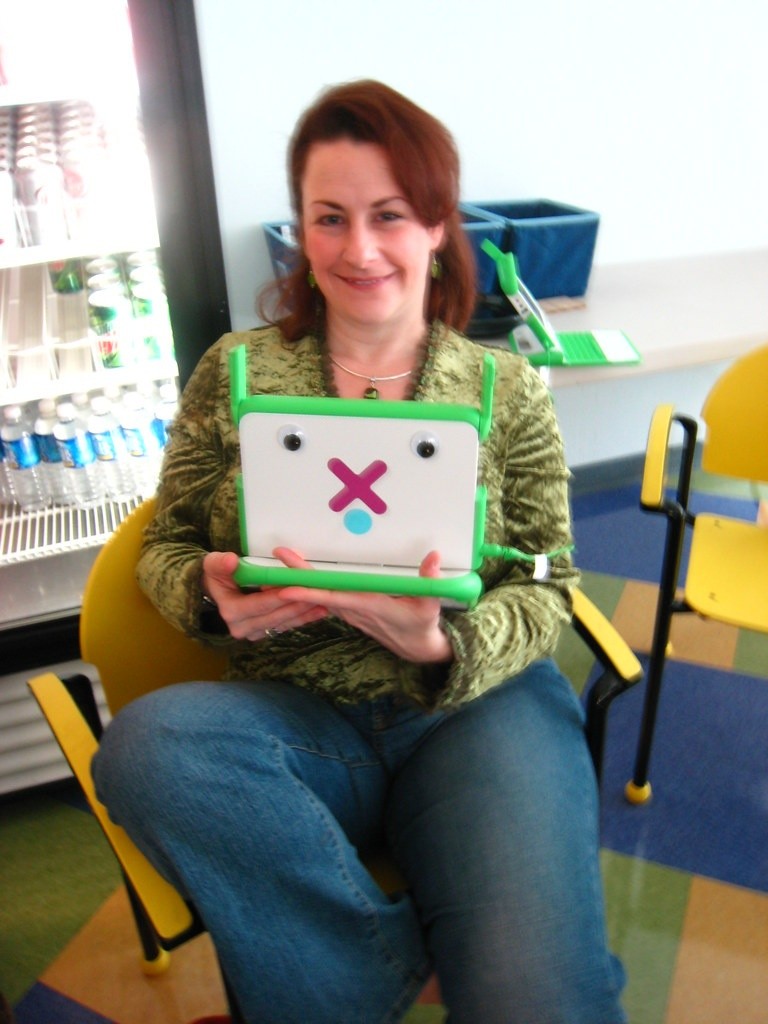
[88,77,633,1024]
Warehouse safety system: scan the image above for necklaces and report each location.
[328,355,412,399]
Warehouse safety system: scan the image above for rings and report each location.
[265,627,282,638]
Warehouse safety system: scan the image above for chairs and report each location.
[625,346,768,804]
[26,493,646,980]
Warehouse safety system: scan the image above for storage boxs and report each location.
[261,197,602,316]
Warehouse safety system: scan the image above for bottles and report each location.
[0,371,178,512]
[0,100,105,248]
